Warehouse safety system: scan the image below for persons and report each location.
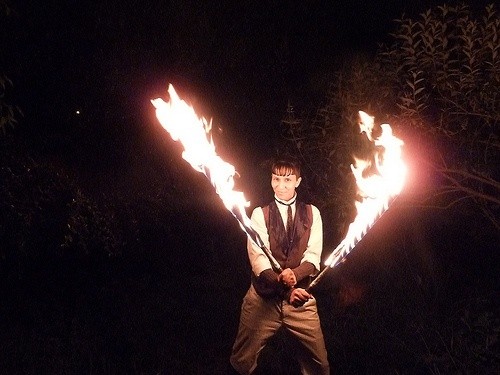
[227,156,331,375]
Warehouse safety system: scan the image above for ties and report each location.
[274,197,297,246]
